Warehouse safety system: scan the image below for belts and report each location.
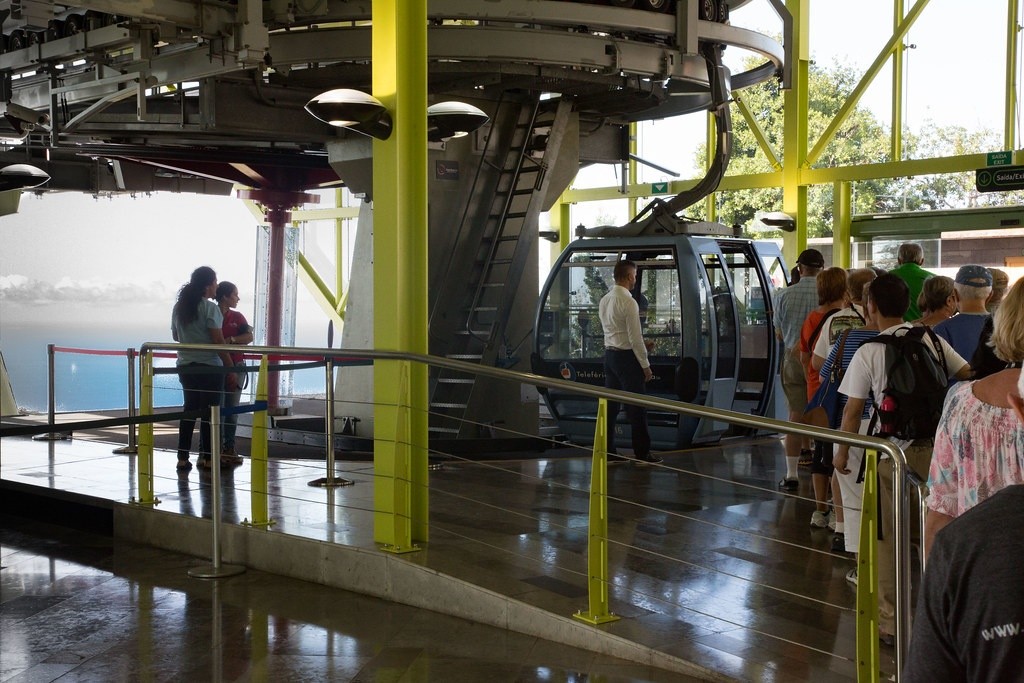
[607,346,623,351]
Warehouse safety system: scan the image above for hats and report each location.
[954,264,993,287]
[796,248,825,267]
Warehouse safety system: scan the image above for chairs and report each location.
[632,290,648,323]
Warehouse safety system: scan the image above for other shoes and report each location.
[832,533,847,545]
[877,628,894,645]
[221,451,243,462]
[177,460,192,468]
[779,477,798,489]
[846,567,857,585]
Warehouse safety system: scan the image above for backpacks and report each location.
[858,324,950,440]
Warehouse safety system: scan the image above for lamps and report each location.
[0,164,52,193]
[760,212,796,232]
[304,88,393,141]
[426,100,489,140]
[538,227,559,244]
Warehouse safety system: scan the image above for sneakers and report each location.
[810,529,830,540]
[606,456,629,464]
[636,454,662,465]
[811,512,828,526]
[828,512,837,529]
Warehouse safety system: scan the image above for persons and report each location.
[887,242,937,321]
[170,265,232,470]
[832,272,975,645]
[899,275,1024,683]
[773,248,889,530]
[918,264,1008,380]
[196,281,254,465]
[599,260,664,466]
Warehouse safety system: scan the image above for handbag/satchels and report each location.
[799,329,852,428]
